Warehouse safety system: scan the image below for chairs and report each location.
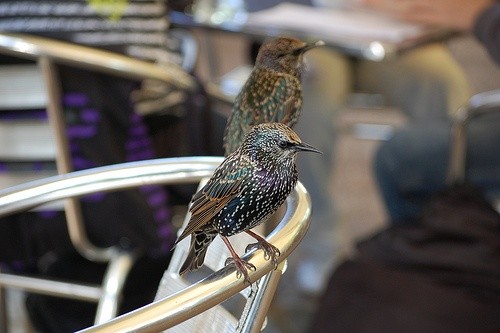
[1,153,313,333]
[0,31,211,266]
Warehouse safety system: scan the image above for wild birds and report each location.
[224,34,327,157]
[167,121,326,284]
